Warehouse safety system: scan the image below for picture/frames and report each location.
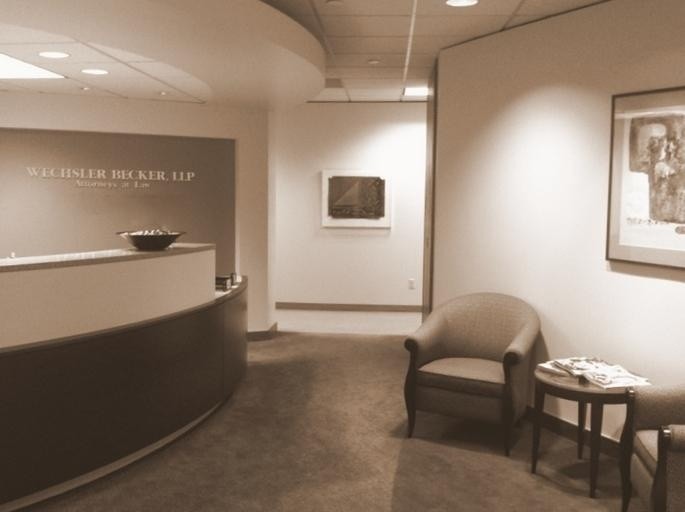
[604,83,685,271]
[321,168,395,230]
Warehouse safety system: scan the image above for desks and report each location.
[531,363,653,497]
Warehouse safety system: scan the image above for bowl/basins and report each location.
[116,231,187,251]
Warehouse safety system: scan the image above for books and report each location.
[537,356,652,390]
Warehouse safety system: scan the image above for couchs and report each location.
[404,292,541,455]
[618,386,685,512]
